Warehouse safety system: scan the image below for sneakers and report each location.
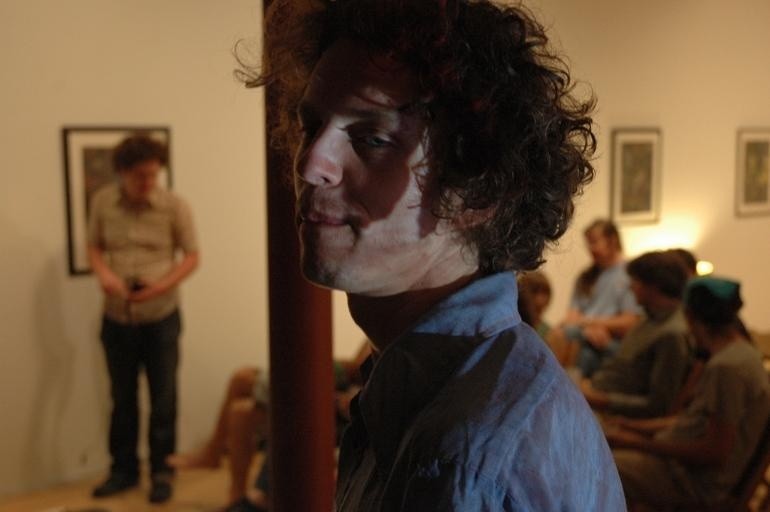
[92,471,139,500]
[148,475,173,504]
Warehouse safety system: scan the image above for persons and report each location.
[575,252,693,422]
[609,276,769,511]
[516,269,567,367]
[669,248,752,412]
[87,134,199,504]
[258,0,627,512]
[165,325,378,511]
[558,217,644,378]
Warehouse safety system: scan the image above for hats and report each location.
[686,275,743,321]
